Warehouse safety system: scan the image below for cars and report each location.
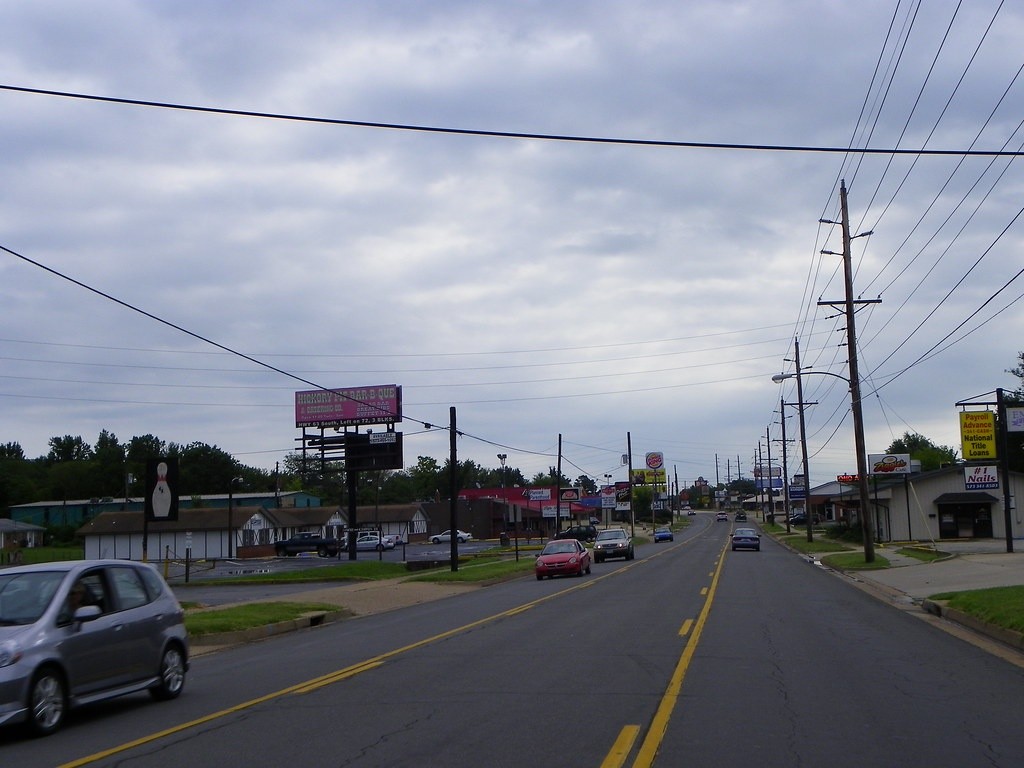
[687,509,696,516]
[1,556,191,741]
[715,511,728,522]
[342,530,402,553]
[428,529,473,544]
[654,527,674,543]
[729,528,763,553]
[589,516,600,525]
[535,539,592,577]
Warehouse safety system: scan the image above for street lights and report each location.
[496,453,507,533]
[772,372,876,563]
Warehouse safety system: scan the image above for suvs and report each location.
[734,509,747,522]
[593,529,635,563]
[554,525,597,543]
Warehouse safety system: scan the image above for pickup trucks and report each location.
[274,531,346,559]
